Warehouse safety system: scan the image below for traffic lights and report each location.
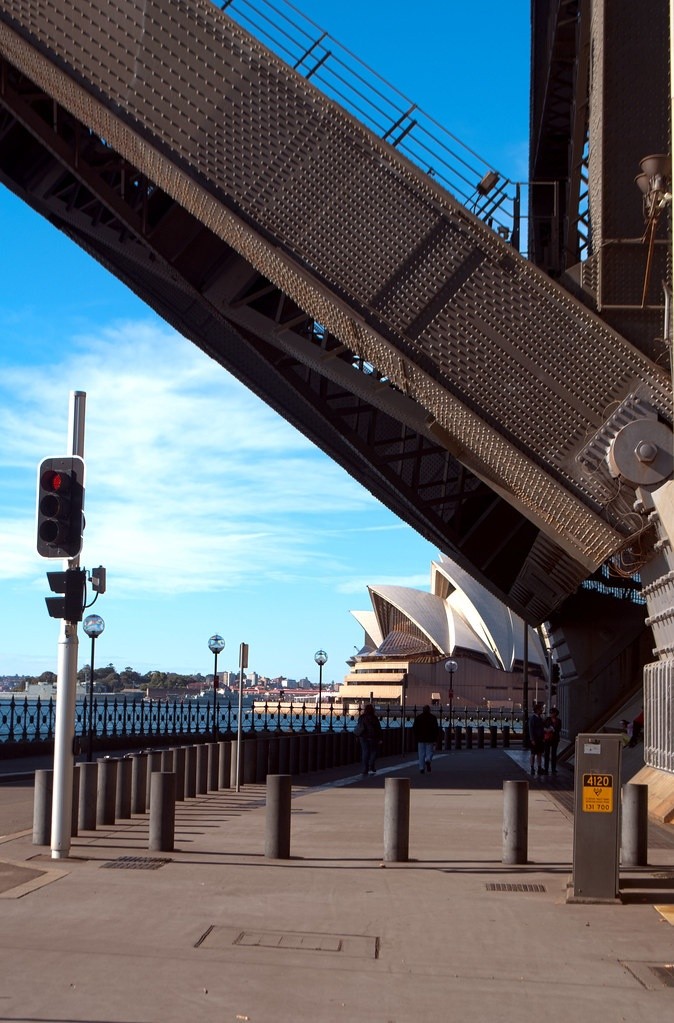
[36,454,86,559]
[44,567,83,622]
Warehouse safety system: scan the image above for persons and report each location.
[355,704,384,774]
[528,703,562,776]
[618,705,644,748]
[412,705,439,774]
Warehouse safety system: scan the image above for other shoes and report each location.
[425,761,431,772]
[420,769,425,773]
[552,768,558,774]
[543,768,548,775]
[362,771,368,774]
[370,767,376,772]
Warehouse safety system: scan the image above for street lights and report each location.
[82,614,105,762]
[444,660,458,732]
[314,650,329,735]
[208,635,225,744]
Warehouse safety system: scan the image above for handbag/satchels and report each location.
[352,717,365,737]
[544,732,553,741]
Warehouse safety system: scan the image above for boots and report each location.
[538,766,543,774]
[531,766,535,774]
